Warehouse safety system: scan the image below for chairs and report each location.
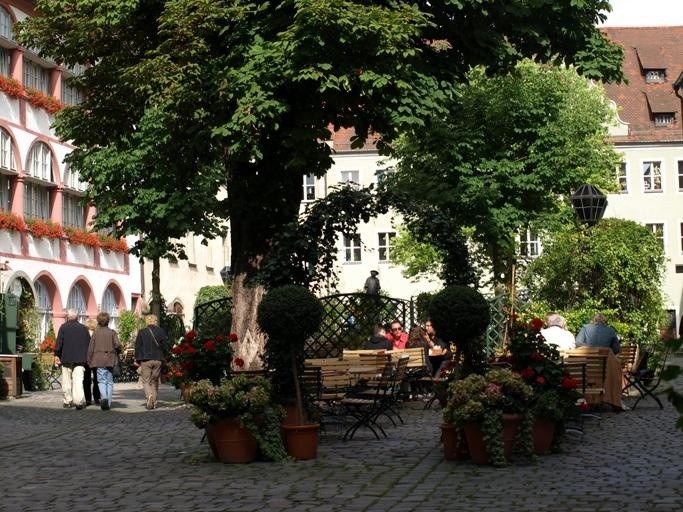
[33,351,62,391]
[111,347,140,383]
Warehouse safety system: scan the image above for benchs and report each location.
[216,338,675,443]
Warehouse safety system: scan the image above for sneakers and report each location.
[100,399,108,411]
[63,403,72,409]
[75,405,84,411]
[147,394,154,410]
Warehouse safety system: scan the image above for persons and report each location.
[134,314,167,409]
[86,312,120,410]
[364,319,452,402]
[83,319,101,404]
[364,270,381,302]
[531,310,619,351]
[54,309,90,409]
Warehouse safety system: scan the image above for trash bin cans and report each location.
[22,352,38,391]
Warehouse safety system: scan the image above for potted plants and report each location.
[163,282,331,465]
[431,281,591,469]
